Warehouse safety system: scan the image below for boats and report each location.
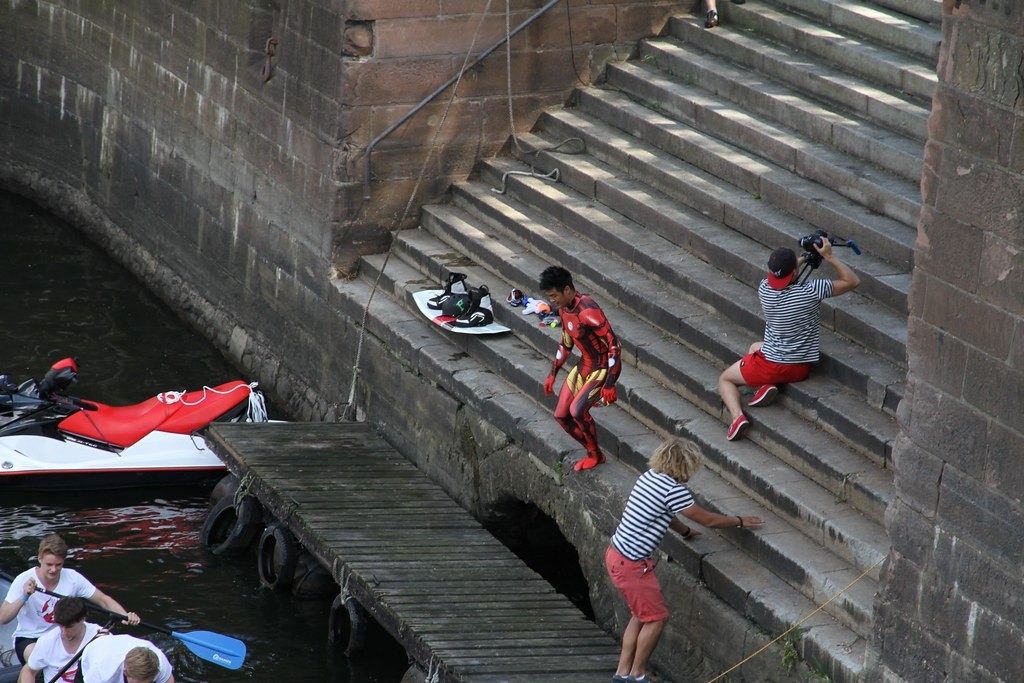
[0,569,47,683]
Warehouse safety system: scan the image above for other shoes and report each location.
[625,674,655,683]
[612,674,627,683]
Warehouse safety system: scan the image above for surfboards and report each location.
[411,288,512,335]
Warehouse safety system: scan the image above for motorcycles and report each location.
[0,357,290,494]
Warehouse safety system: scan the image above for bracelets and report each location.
[21,594,28,601]
[736,516,743,528]
[682,527,690,537]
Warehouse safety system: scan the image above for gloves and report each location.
[544,375,555,397]
[600,385,617,406]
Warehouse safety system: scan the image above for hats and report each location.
[767,246,796,290]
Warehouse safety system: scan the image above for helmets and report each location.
[442,295,471,316]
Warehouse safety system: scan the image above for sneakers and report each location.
[747,384,778,407]
[726,414,749,441]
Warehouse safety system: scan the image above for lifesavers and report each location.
[256,522,300,591]
[327,590,365,659]
[199,491,265,560]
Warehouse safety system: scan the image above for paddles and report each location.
[35,585,247,671]
[47,612,121,683]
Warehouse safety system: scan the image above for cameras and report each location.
[798,228,835,259]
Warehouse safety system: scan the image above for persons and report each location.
[0,534,141,665]
[605,437,765,683]
[21,598,113,683]
[718,236,861,440]
[74,635,175,683]
[704,0,719,28]
[539,266,622,471]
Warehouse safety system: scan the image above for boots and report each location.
[426,272,467,309]
[452,285,494,327]
